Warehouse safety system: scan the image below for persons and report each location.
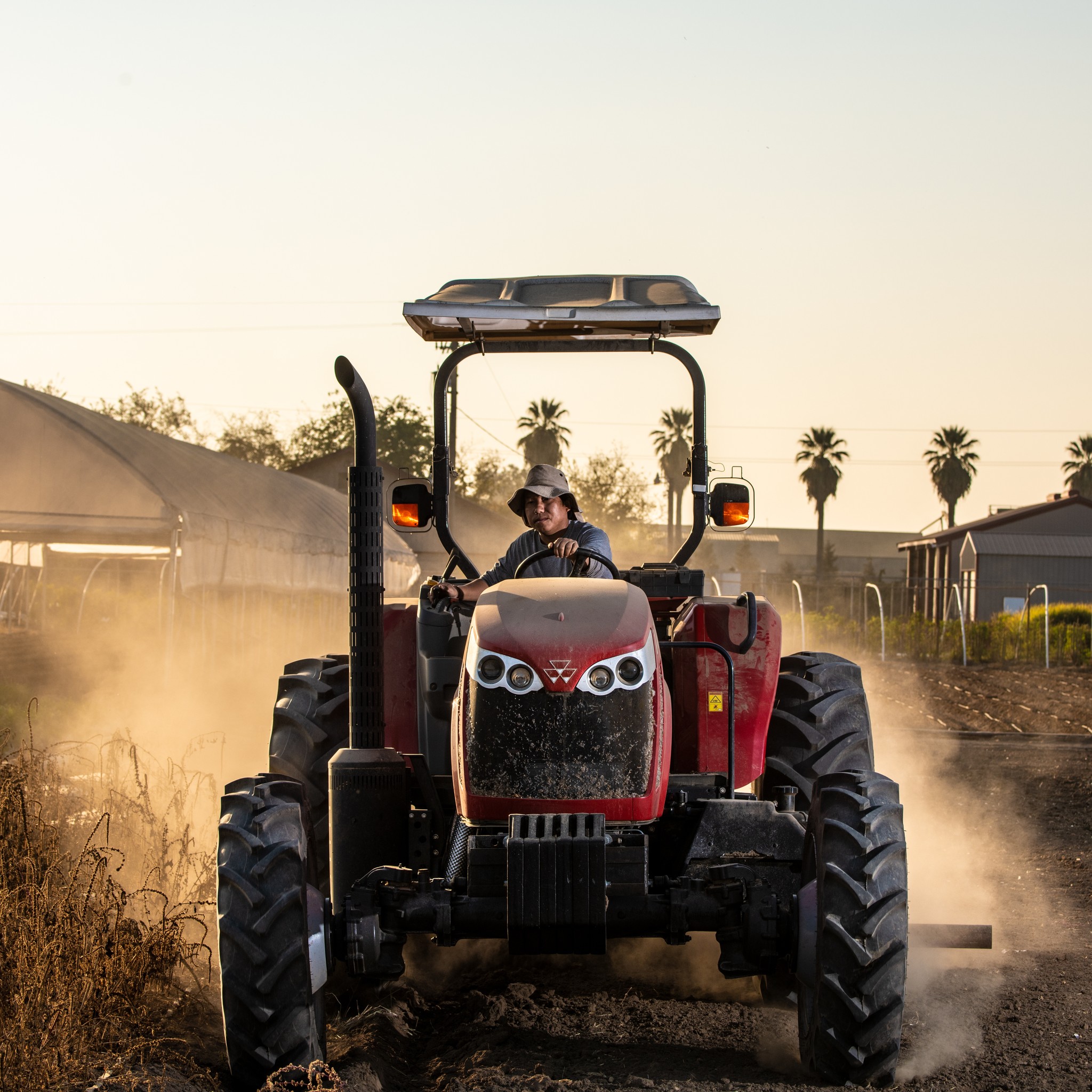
[428,464,613,604]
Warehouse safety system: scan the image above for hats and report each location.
[507,464,584,527]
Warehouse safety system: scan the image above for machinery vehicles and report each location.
[216,278,909,1092]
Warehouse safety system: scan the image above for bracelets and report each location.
[454,586,464,600]
